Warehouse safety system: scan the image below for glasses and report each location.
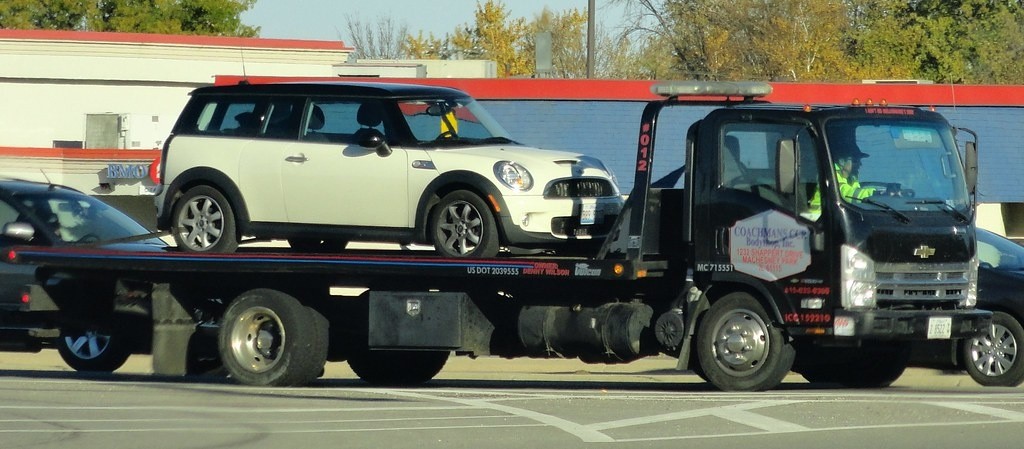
[844,159,860,163]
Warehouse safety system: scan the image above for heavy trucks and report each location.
[0,72,1000,392]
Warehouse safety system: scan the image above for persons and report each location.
[809,143,906,218]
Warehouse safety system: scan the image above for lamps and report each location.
[99,182,115,192]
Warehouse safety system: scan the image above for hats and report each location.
[832,143,870,160]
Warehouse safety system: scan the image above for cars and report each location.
[1,177,172,372]
[960,226,1024,387]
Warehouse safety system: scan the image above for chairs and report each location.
[354,103,384,143]
[292,105,327,140]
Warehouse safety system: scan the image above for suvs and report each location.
[151,73,623,260]
